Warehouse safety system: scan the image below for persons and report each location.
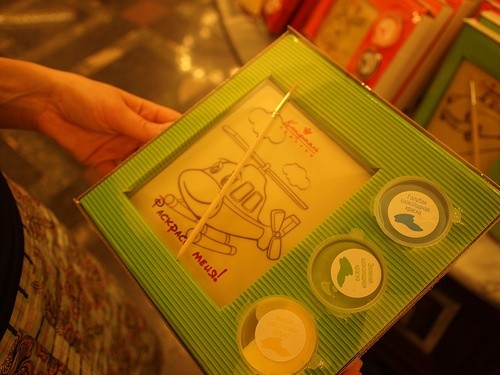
[0,55,366,375]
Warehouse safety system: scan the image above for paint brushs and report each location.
[469,79,482,174]
[174,82,295,263]
